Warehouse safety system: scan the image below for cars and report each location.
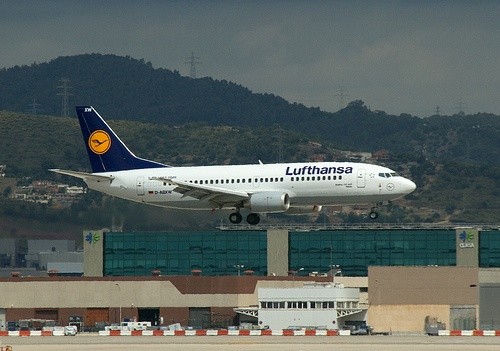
[351,328,367,335]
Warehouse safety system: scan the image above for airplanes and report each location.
[46,106,418,225]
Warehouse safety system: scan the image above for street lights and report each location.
[115,282,122,327]
